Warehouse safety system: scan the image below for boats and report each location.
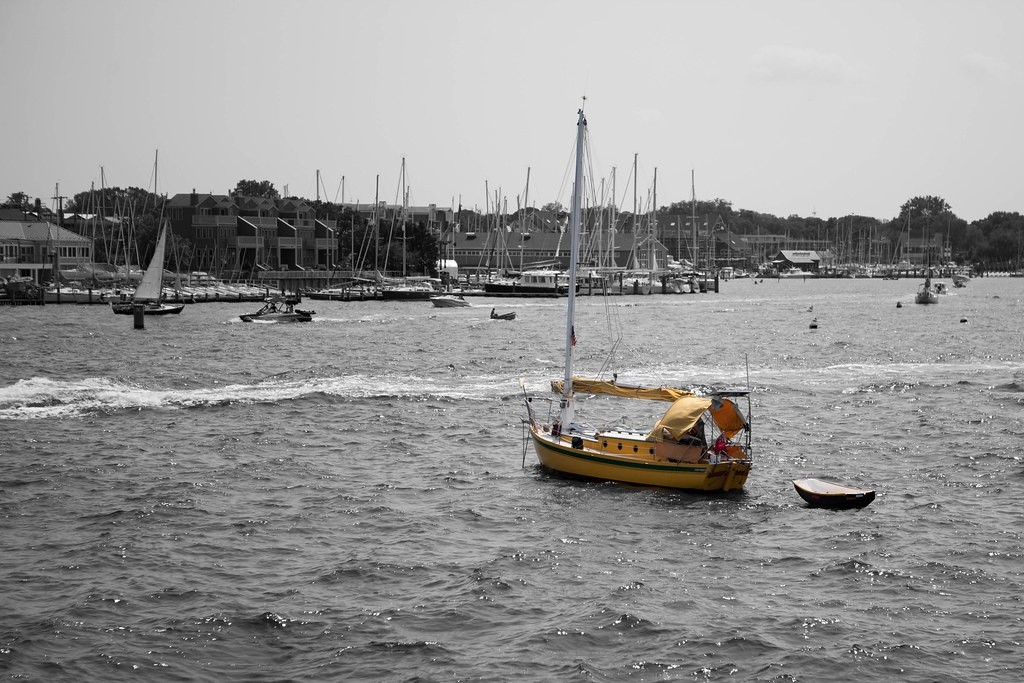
[430,294,471,307]
[791,477,877,512]
[490,312,517,320]
[238,293,317,324]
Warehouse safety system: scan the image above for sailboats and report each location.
[6,149,976,305]
[516,95,759,492]
[110,218,186,314]
